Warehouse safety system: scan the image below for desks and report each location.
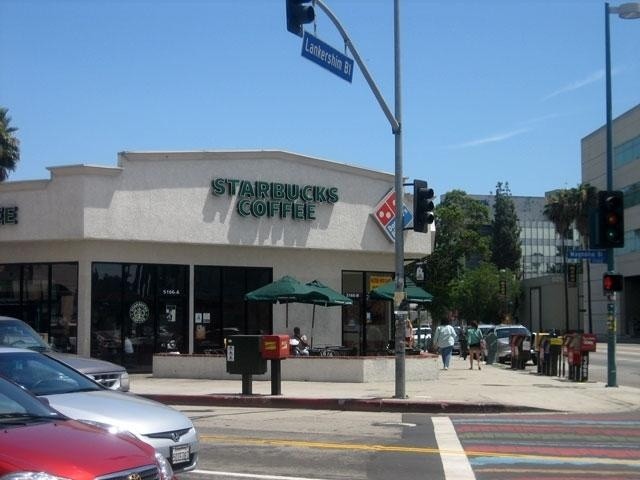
[314,346,355,357]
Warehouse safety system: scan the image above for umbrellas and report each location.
[303,279,354,350]
[364,275,434,305]
[244,274,331,329]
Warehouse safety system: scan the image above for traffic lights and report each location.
[597,191,623,250]
[285,0,317,40]
[603,273,623,295]
[412,179,436,233]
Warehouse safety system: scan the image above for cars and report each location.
[0,313,200,480]
[411,323,538,366]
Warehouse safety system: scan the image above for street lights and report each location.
[604,1,640,387]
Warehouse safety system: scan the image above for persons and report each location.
[114,335,135,358]
[466,320,484,372]
[402,317,415,348]
[432,316,458,370]
[460,328,470,360]
[292,326,310,357]
